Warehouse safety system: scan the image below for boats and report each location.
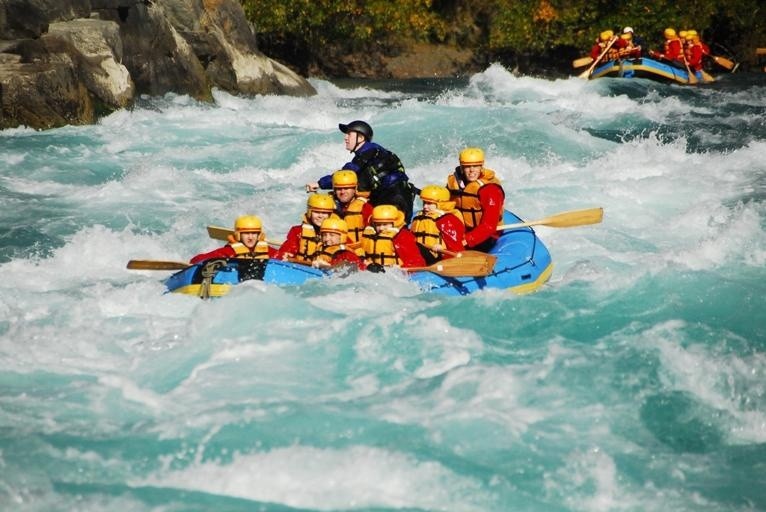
[166,210,553,299]
[588,57,716,86]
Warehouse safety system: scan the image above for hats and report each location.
[623,27,634,33]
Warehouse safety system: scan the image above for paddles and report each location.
[709,55,734,69]
[423,244,497,275]
[497,207,603,231]
[406,255,486,276]
[684,57,698,84]
[126,260,193,270]
[578,34,619,81]
[207,225,283,247]
[756,48,766,54]
[573,45,641,68]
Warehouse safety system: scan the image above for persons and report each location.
[303,120,413,224]
[188,213,280,266]
[361,203,427,268]
[326,169,374,246]
[274,192,342,265]
[443,146,506,254]
[307,215,368,271]
[590,26,712,74]
[408,183,465,267]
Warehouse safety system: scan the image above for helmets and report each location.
[601,31,612,40]
[621,34,631,39]
[664,28,677,39]
[420,185,451,208]
[307,194,335,219]
[604,30,614,35]
[332,170,359,194]
[234,216,265,242]
[338,120,373,141]
[459,147,485,166]
[686,30,698,41]
[320,218,348,243]
[373,205,401,226]
[679,31,686,38]
